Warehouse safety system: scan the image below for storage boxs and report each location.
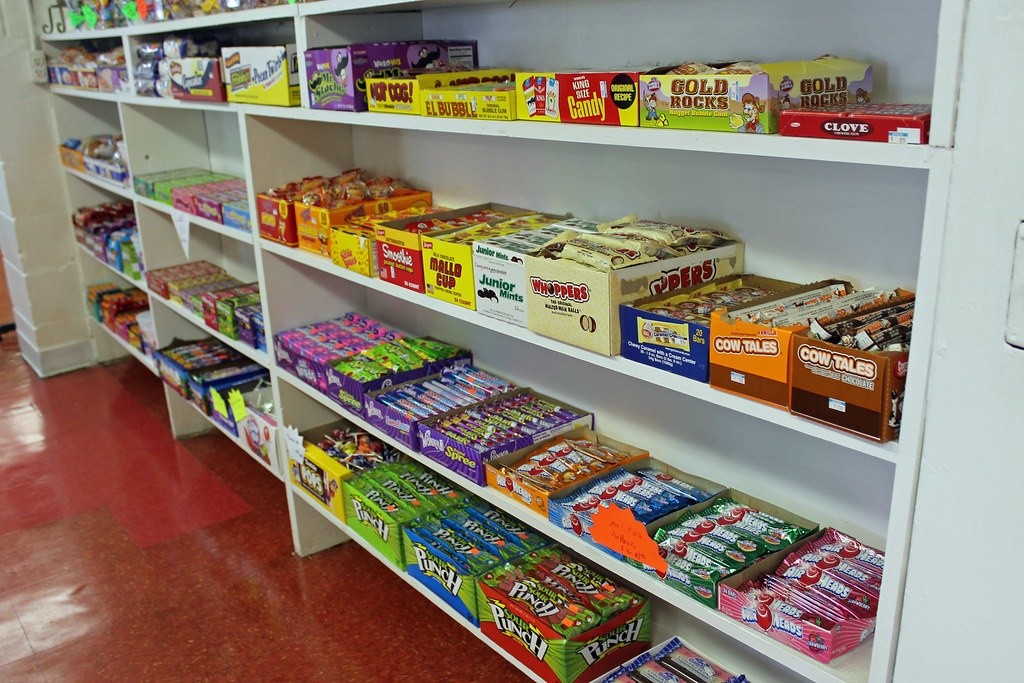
[59,45,935,683]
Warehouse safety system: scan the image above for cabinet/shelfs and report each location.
[41,0,968,683]
